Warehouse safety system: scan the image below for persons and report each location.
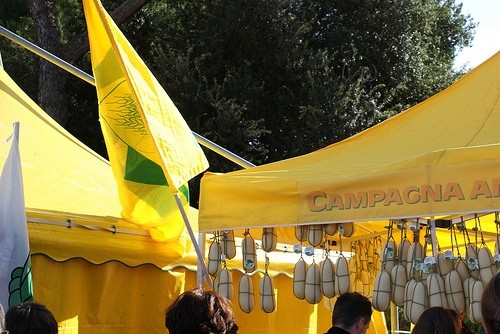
[165,288,242,334]
[479,272,500,334]
[322,292,373,334]
[410,307,462,334]
[5,302,59,334]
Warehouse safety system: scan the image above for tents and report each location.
[1,62,389,334]
[198,50,499,293]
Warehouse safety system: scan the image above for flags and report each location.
[83,1,210,242]
[0,120,33,315]
